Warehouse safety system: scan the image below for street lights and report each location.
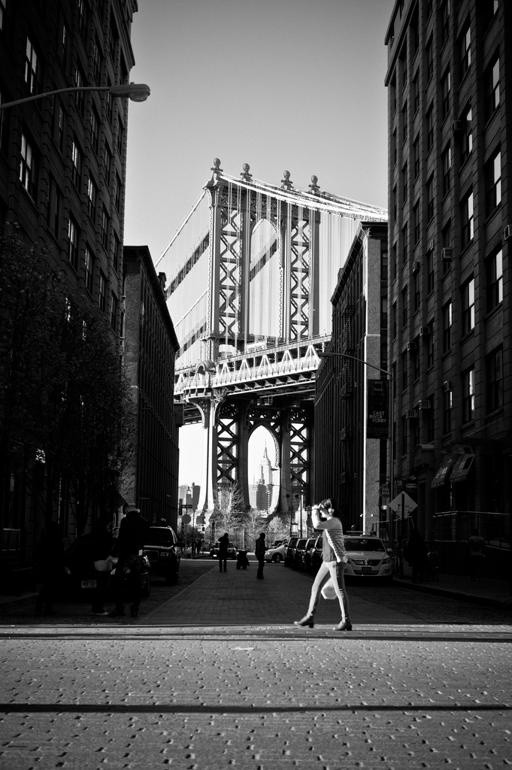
[317,349,396,531]
[1,82,149,108]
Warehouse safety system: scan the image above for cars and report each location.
[263,535,394,583]
[142,523,182,586]
[211,542,247,559]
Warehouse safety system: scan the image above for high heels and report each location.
[291,614,360,632]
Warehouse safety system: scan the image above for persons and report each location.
[89,503,149,617]
[254,532,268,581]
[407,528,428,583]
[294,497,353,631]
[218,533,229,573]
[469,528,487,577]
[191,539,202,554]
